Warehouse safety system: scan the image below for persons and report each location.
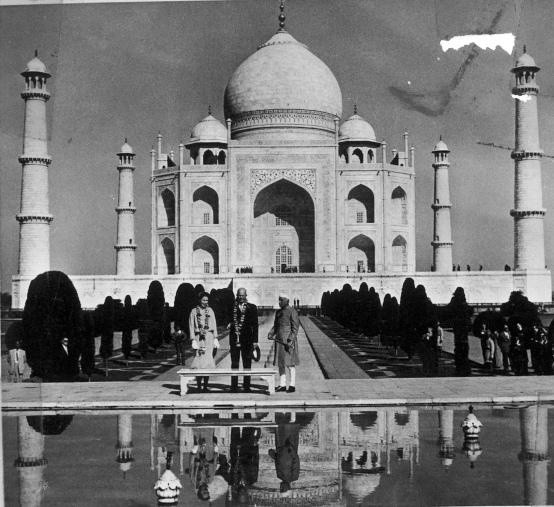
[188,292,219,392]
[479,321,554,376]
[420,321,443,358]
[172,325,188,366]
[267,295,299,393]
[189,412,302,502]
[7,339,28,384]
[53,337,74,383]
[229,287,258,393]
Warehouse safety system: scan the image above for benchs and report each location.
[174,412,281,432]
[176,368,281,399]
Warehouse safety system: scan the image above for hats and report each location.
[280,490,291,498]
[279,292,290,299]
[250,346,261,362]
[250,427,261,443]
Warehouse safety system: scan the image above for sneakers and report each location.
[231,386,241,392]
[244,386,251,392]
[195,388,201,393]
[286,386,295,393]
[202,388,210,393]
[275,386,286,392]
